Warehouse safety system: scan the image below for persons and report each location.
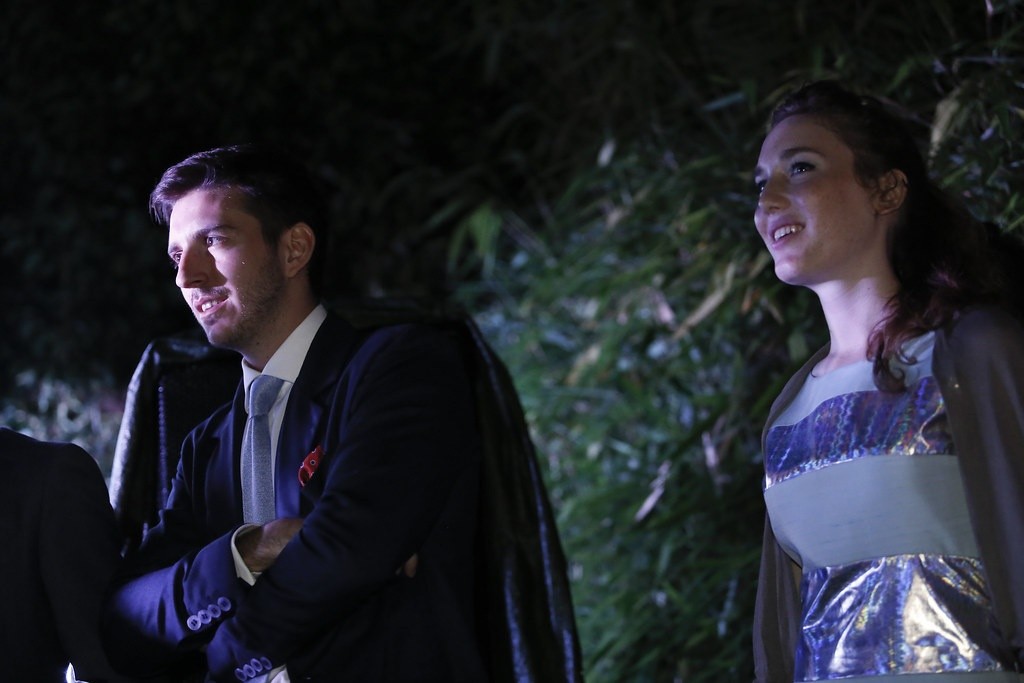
[755,77,1024,682]
[1,426,122,683]
[109,141,494,683]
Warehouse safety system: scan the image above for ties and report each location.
[240,375,285,523]
